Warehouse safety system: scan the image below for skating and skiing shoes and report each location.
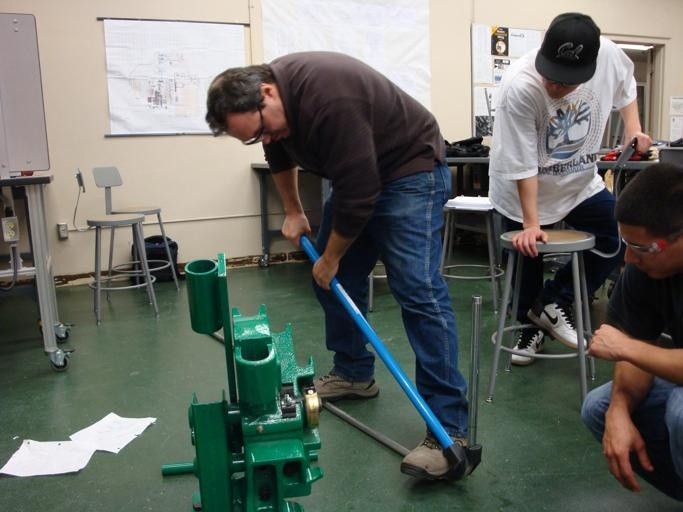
[314,370,380,404]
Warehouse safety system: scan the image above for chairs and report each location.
[92,165,181,302]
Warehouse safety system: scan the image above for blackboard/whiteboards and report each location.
[0,13,53,186]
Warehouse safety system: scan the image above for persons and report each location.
[202,52,468,482]
[579,161,682,503]
[487,12,653,364]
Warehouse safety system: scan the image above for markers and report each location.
[9,171,34,178]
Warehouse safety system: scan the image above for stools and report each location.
[439,196,597,414]
[88,215,159,327]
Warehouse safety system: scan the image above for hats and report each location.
[533,10,601,87]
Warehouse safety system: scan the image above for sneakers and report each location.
[510,320,546,366]
[526,297,589,351]
[400,431,470,480]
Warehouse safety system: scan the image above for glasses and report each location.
[241,108,265,147]
[544,78,581,88]
[620,231,682,257]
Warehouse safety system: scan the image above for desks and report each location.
[251,157,657,268]
[2,173,79,371]
